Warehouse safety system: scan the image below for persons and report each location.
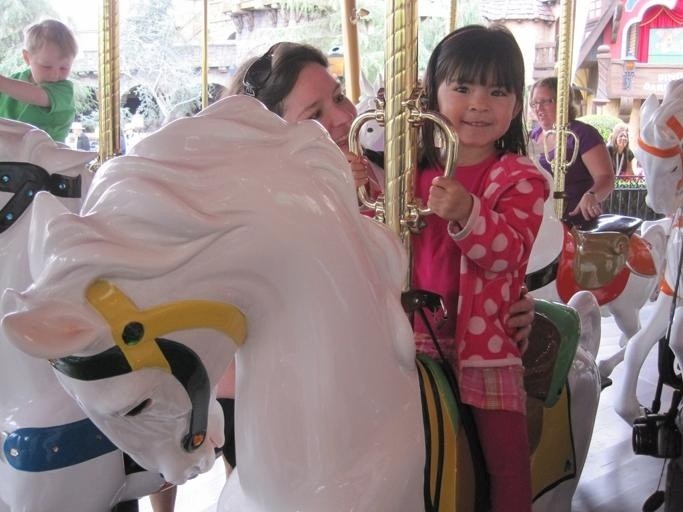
[524,76,614,228]
[120,85,236,512]
[71,121,91,151]
[219,42,358,156]
[0,19,77,145]
[347,25,550,512]
[606,124,636,176]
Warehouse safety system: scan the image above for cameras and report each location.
[632,414,678,458]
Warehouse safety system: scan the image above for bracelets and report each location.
[583,189,603,204]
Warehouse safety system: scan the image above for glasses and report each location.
[529,97,556,109]
[243,42,303,97]
